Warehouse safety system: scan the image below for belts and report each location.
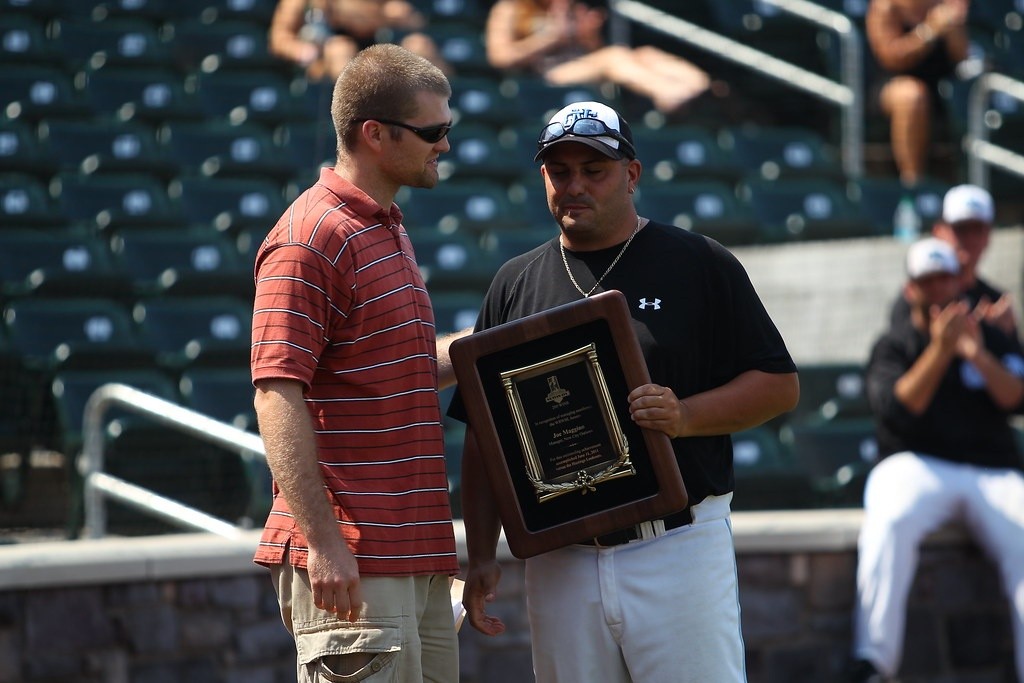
[573,506,696,546]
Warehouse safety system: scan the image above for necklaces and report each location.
[560,215,641,298]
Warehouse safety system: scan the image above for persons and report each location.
[852,0,1024,683]
[444,100,801,683]
[250,46,479,683]
[269,0,611,100]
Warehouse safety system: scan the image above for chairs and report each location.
[1,2,1024,536]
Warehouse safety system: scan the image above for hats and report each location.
[534,101,634,162]
[941,184,995,225]
[905,238,962,282]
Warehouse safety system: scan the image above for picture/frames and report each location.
[448,289,689,560]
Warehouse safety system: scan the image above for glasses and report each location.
[355,117,453,143]
[537,118,637,155]
